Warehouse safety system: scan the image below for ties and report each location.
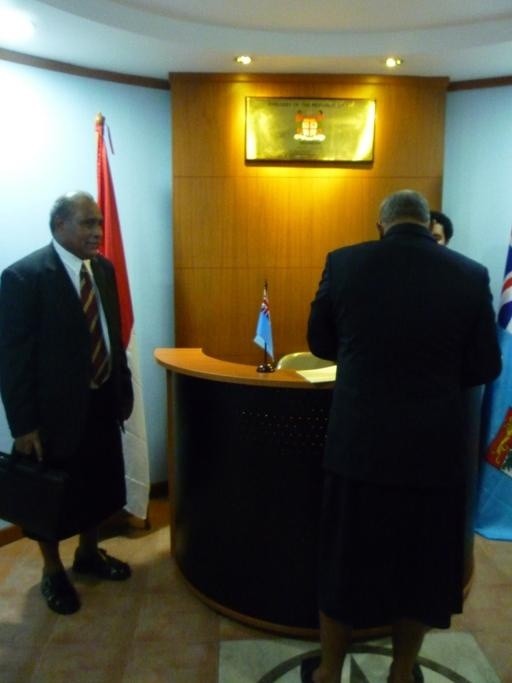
[78,262,110,388]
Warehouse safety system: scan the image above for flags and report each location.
[494,223,511,334]
[251,279,275,362]
[93,113,138,352]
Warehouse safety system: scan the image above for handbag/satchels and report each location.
[0,451,87,542]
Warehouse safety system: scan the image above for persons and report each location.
[296,187,504,681]
[2,189,136,617]
[424,209,454,248]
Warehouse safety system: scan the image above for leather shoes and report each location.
[73,544,134,581]
[41,560,81,614]
[300,655,424,683]
